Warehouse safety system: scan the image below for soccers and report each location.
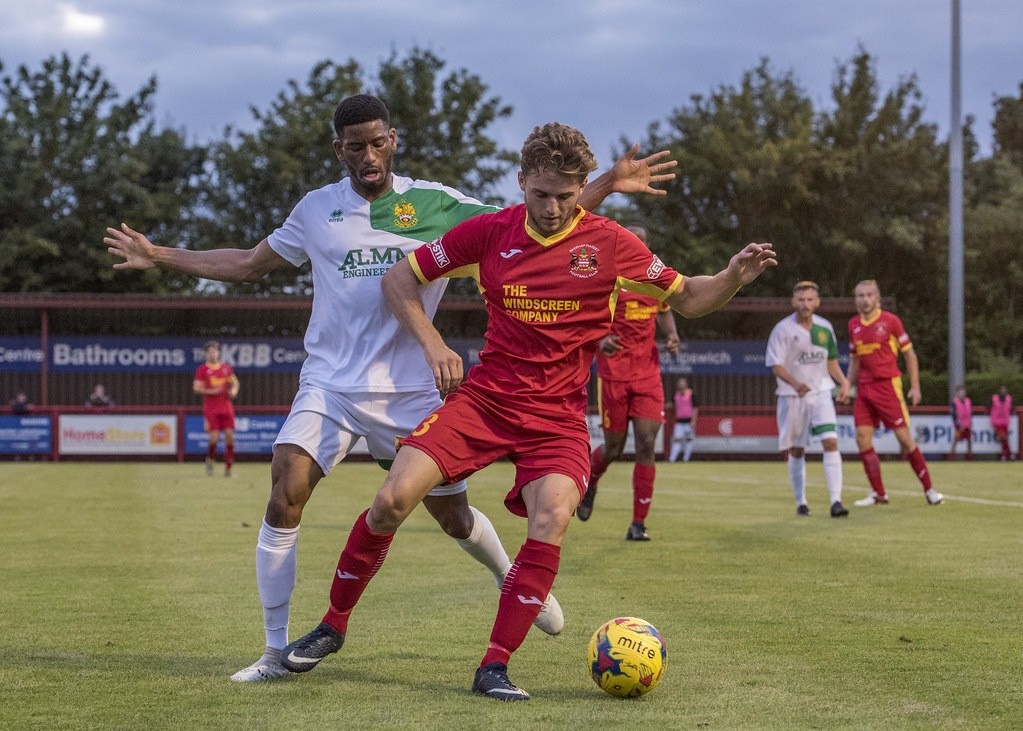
[585,615,668,699]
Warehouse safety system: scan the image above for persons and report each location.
[984,385,1016,462]
[842,278,945,506]
[279,122,777,701]
[577,215,679,539]
[9,392,34,413]
[193,340,241,477]
[86,384,115,408]
[104,94,677,681]
[668,377,699,464]
[765,281,850,517]
[949,384,973,462]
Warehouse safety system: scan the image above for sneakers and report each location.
[626,522,650,541]
[470,662,532,702]
[578,486,596,520]
[280,622,345,673]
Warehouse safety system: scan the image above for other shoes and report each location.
[853,491,888,507]
[925,488,944,505]
[832,502,848,516]
[798,505,809,516]
[533,593,564,636]
[229,653,299,687]
